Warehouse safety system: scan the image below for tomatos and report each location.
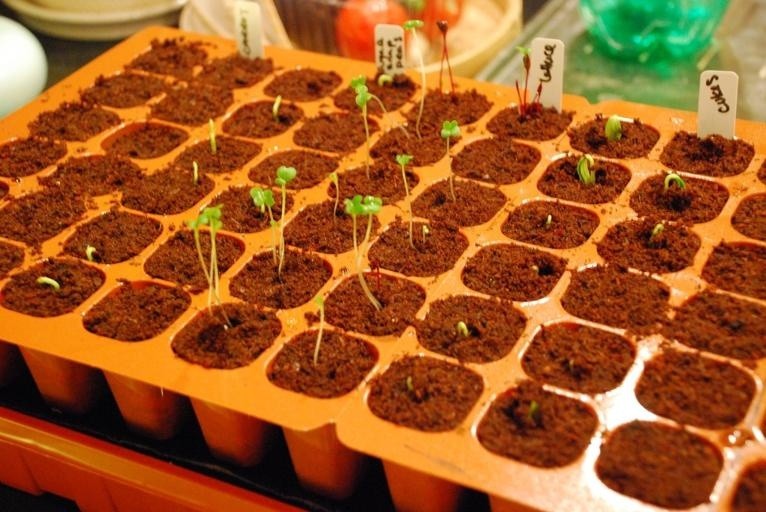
[398,0,464,42]
[336,0,412,62]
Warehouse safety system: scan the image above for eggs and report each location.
[0,15,50,118]
